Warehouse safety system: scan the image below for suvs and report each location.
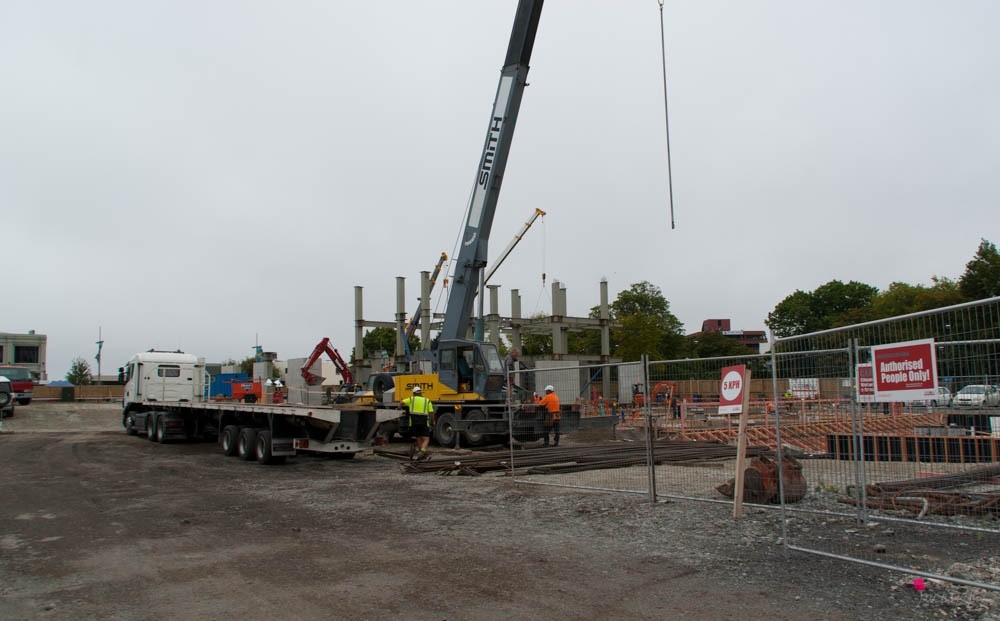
[0,376,14,417]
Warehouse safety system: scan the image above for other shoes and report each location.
[542,443,548,446]
[551,444,558,447]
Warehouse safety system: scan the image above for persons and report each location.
[400,387,436,451]
[783,390,794,411]
[504,349,519,378]
[765,402,775,413]
[534,385,561,447]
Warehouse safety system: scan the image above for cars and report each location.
[952,385,1000,409]
[912,387,953,408]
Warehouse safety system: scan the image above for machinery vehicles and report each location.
[631,381,678,406]
[352,0,676,450]
[301,338,361,404]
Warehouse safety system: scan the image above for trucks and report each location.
[0,362,33,405]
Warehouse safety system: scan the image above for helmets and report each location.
[413,387,421,393]
[544,385,553,391]
[788,390,792,394]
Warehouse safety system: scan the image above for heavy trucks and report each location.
[118,350,407,464]
